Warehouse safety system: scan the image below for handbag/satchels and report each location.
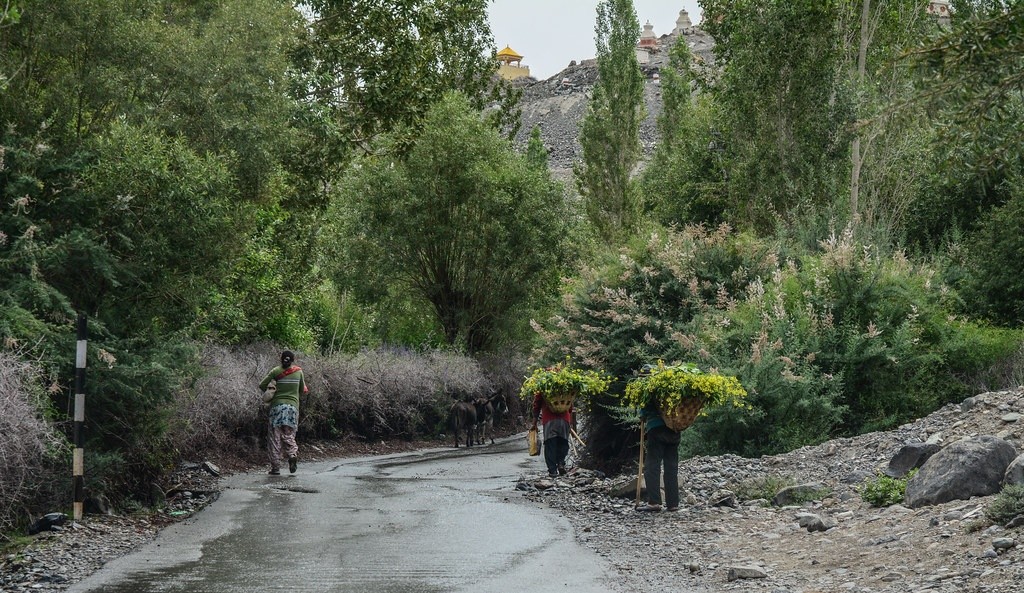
[526,430,541,456]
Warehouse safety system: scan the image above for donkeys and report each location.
[446,387,508,448]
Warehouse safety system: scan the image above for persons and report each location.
[637,364,682,512]
[259,351,309,475]
[529,365,578,477]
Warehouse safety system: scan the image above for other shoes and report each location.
[549,471,558,477]
[288,455,297,473]
[559,467,567,476]
[645,504,661,510]
[666,507,679,511]
[269,469,280,475]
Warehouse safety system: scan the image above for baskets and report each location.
[655,397,706,430]
[541,387,580,414]
